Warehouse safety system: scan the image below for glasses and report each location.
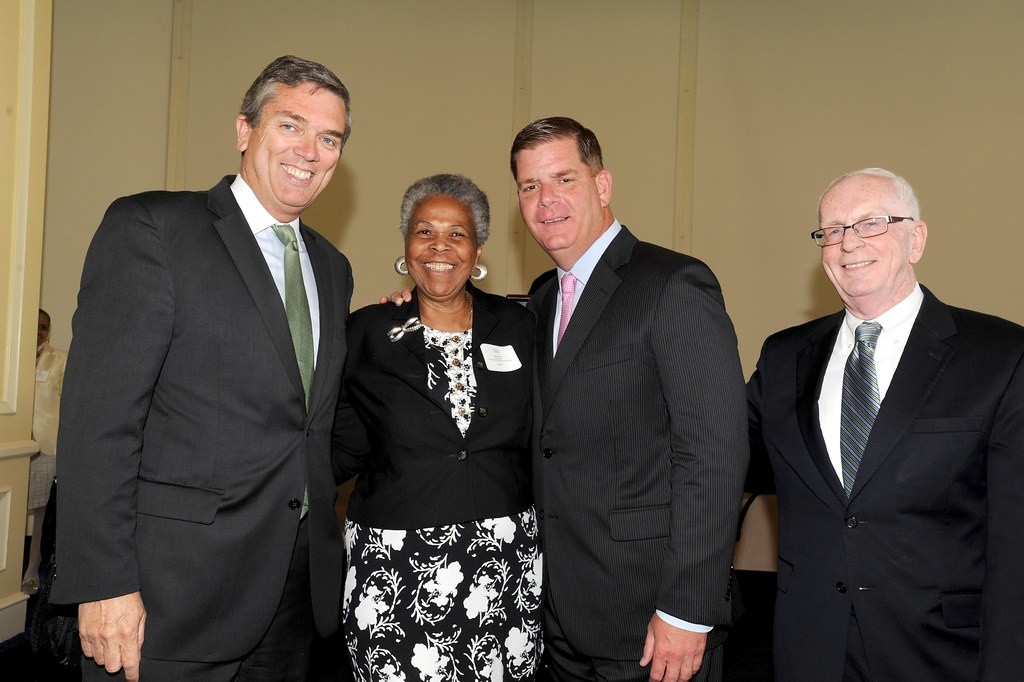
[811,215,914,247]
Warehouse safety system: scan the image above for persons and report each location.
[21,304,72,595]
[330,174,546,682]
[745,166,1023,682]
[45,55,355,682]
[378,115,752,682]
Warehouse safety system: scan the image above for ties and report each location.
[271,224,314,520]
[557,274,577,347]
[841,322,882,500]
